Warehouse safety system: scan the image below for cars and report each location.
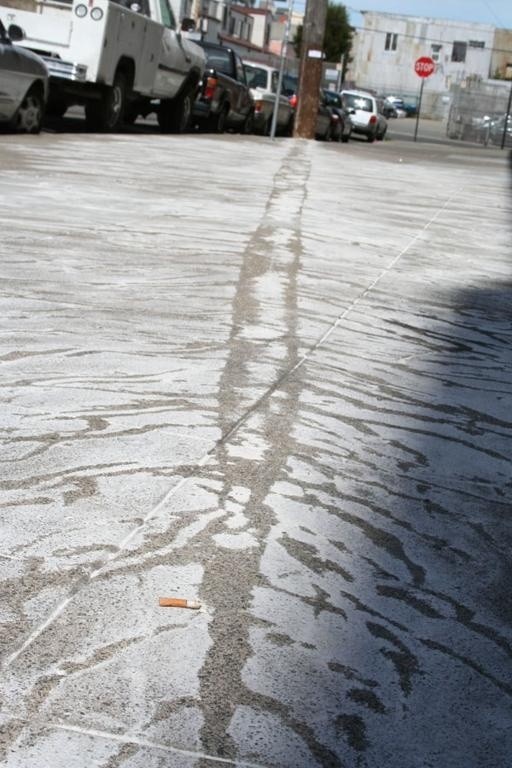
[250,71,333,141]
[205,60,294,134]
[321,88,356,142]
[378,95,416,118]
[1,17,50,135]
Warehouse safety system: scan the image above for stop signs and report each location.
[415,57,435,78]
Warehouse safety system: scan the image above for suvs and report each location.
[338,90,387,143]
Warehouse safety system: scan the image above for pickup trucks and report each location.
[0,0,209,130]
[147,38,256,134]
